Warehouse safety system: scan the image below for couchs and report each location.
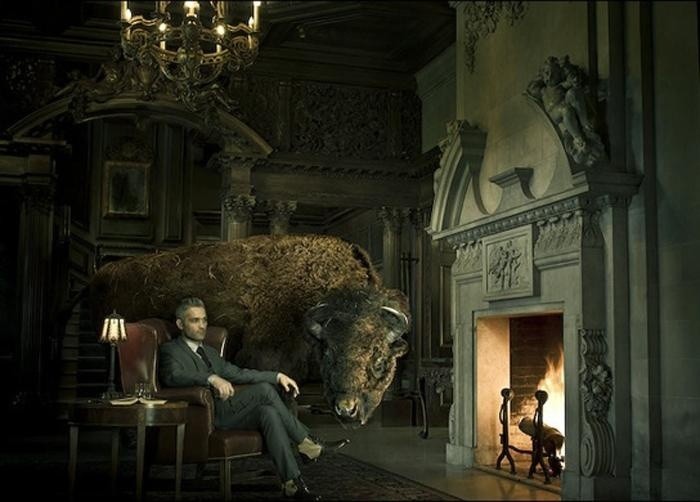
[118,318,295,501]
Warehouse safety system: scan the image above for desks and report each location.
[52,396,187,501]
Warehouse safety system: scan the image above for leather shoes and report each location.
[298,435,351,466]
[282,478,322,501]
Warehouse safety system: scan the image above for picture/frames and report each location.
[99,159,152,223]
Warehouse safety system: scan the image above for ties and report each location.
[197,347,212,369]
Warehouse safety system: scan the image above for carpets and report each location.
[1,444,461,501]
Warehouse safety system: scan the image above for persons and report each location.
[157,298,350,501]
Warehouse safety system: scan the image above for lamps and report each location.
[98,307,122,398]
[114,1,269,134]
